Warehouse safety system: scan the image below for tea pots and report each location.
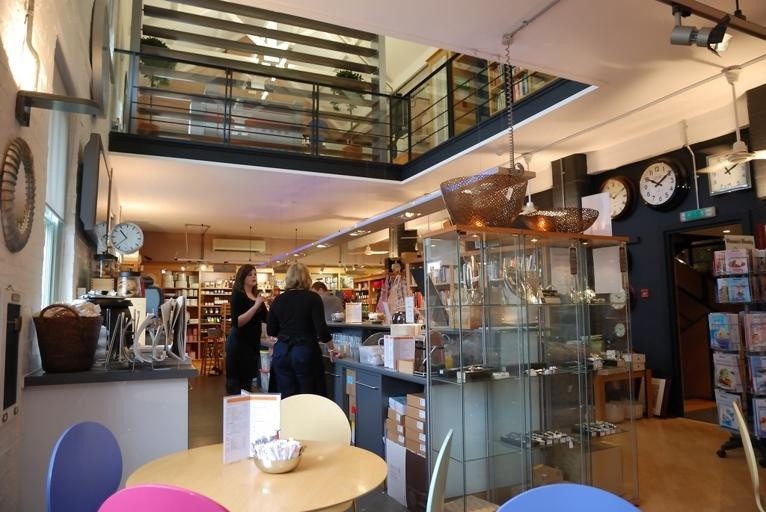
[391,310,406,324]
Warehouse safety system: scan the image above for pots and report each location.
[89,292,133,349]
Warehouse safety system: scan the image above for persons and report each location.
[226,264,273,396]
[267,263,346,400]
[311,281,345,321]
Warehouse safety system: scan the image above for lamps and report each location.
[669,4,733,61]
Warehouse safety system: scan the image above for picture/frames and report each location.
[639,374,672,418]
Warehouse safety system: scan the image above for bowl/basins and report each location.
[252,456,303,474]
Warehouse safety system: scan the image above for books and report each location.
[707,232,766,440]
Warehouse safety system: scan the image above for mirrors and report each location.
[0,135,38,256]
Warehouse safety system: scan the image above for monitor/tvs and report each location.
[77,132,109,246]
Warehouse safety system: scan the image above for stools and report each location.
[593,363,654,423]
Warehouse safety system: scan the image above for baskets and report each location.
[440,174,527,233]
[520,198,600,234]
[33,304,105,374]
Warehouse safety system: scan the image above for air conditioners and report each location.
[213,239,267,253]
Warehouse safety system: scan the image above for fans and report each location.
[399,213,446,240]
[344,234,390,256]
[517,151,570,219]
[694,65,766,173]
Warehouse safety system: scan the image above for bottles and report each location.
[359,294,368,317]
[93,254,145,297]
[201,307,223,357]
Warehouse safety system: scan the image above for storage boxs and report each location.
[489,321,543,367]
[592,440,624,500]
[385,335,427,370]
[384,391,425,511]
[342,369,355,443]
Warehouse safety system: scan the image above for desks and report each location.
[124,436,390,510]
[319,355,550,510]
[22,349,200,511]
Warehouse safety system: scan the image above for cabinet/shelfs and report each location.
[421,224,638,510]
[160,266,359,374]
[353,241,546,314]
[487,56,562,119]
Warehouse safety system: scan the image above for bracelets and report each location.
[327,345,337,352]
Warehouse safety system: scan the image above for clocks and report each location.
[599,175,635,222]
[637,157,690,212]
[110,219,144,254]
[706,146,752,199]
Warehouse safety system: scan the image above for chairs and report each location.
[44,418,124,510]
[97,484,228,511]
[730,399,766,510]
[426,428,455,510]
[281,392,353,444]
[495,481,642,511]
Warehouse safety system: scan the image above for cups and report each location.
[162,272,198,306]
[186,319,198,360]
[201,290,232,306]
[229,275,236,288]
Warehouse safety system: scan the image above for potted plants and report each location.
[331,66,369,162]
[139,37,178,140]
